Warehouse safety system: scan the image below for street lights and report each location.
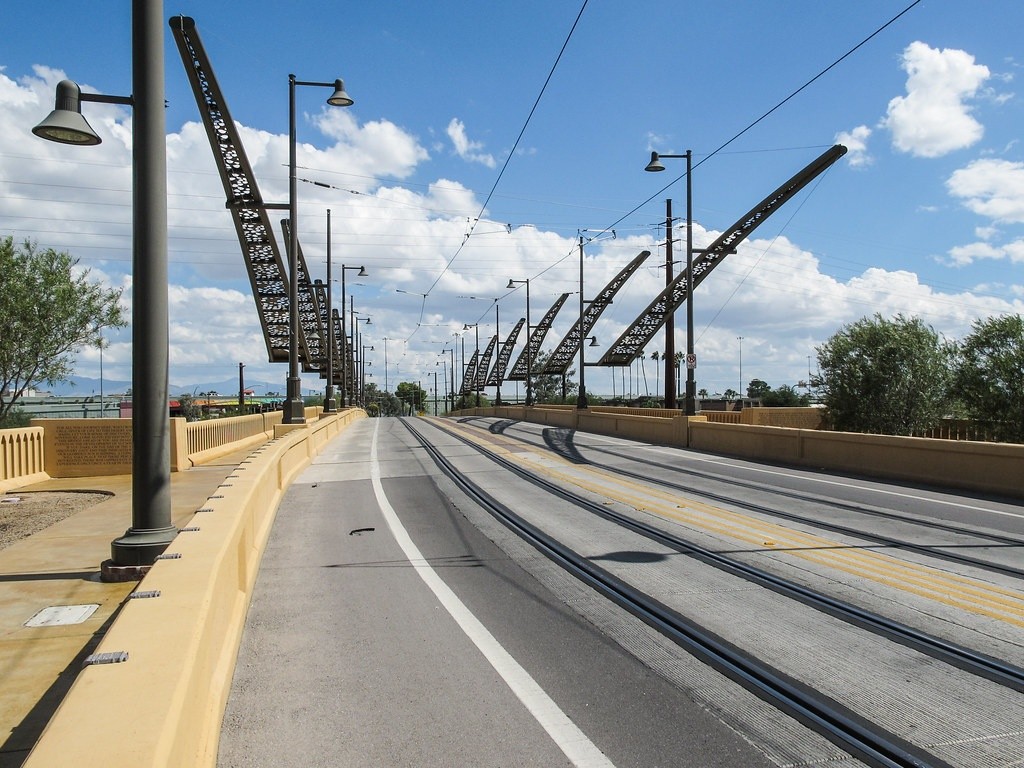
[736,336,744,399]
[354,316,373,410]
[463,323,483,409]
[32,79,182,566]
[340,264,368,408]
[564,234,614,409]
[427,371,438,417]
[807,354,812,392]
[644,149,698,416]
[276,72,355,427]
[441,348,455,409]
[506,279,537,404]
[436,361,448,414]
